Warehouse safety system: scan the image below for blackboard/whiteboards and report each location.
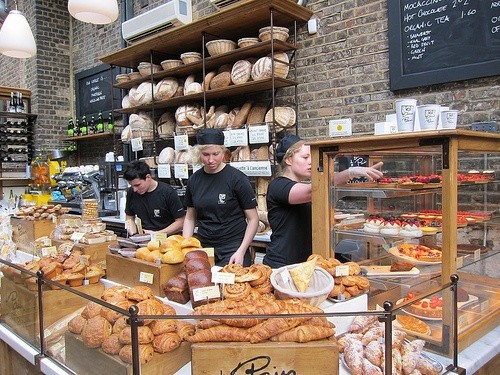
[339,155,369,183]
[387,0,500,92]
[75,64,122,126]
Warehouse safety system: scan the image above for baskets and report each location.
[116,24,297,168]
[270,263,334,306]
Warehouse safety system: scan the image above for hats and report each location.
[274,134,300,163]
[196,128,225,146]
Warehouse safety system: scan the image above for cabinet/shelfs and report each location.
[98,0,313,178]
[311,129,500,273]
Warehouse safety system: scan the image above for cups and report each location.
[21,190,61,208]
[441,110,457,129]
[156,125,175,139]
[417,105,439,130]
[395,99,417,132]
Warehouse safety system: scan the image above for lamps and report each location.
[67,0,119,25]
[0,0,35,60]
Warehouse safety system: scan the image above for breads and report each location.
[307,254,442,375]
[0,205,116,292]
[123,57,271,163]
[68,234,336,365]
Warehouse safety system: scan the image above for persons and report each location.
[182,128,259,268]
[123,161,186,237]
[262,133,383,270]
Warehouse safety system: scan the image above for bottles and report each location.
[68,109,114,138]
[0,91,37,166]
[0,189,26,213]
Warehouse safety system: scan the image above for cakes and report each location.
[363,173,494,318]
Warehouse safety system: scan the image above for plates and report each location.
[122,76,179,109]
[362,265,420,277]
[203,57,272,90]
[389,246,442,266]
[396,298,442,321]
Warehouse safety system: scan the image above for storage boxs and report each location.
[10,217,56,255]
[1,274,110,347]
[63,331,192,375]
[51,236,121,265]
[191,334,343,375]
[105,249,217,297]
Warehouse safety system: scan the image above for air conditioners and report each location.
[121,0,192,45]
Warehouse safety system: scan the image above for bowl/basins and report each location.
[270,263,335,308]
[205,25,289,56]
[265,107,290,134]
[132,125,198,169]
[267,49,290,79]
[116,52,201,83]
